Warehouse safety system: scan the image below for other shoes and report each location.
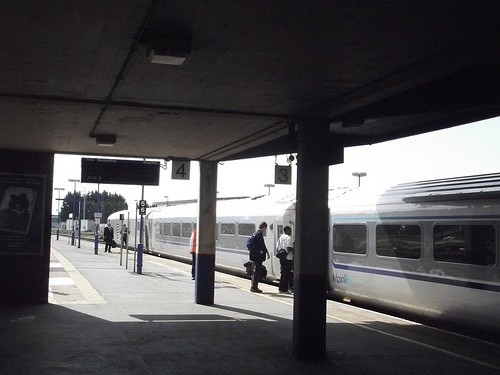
[278,290,292,295]
[250,290,262,293]
[192,277,195,280]
[108,251,111,252]
[105,250,107,252]
[289,288,295,293]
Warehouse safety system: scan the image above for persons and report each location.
[120,224,130,254]
[249,222,271,292]
[103,222,114,253]
[189,230,196,280]
[276,225,295,294]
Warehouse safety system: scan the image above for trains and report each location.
[106,172,499,340]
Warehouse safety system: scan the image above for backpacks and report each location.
[246,235,256,251]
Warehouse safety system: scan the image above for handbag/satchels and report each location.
[244,261,267,279]
[111,240,117,248]
[276,249,287,259]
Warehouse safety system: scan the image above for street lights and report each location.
[54,187,65,230]
[352,173,366,187]
[69,179,80,245]
[264,184,276,196]
